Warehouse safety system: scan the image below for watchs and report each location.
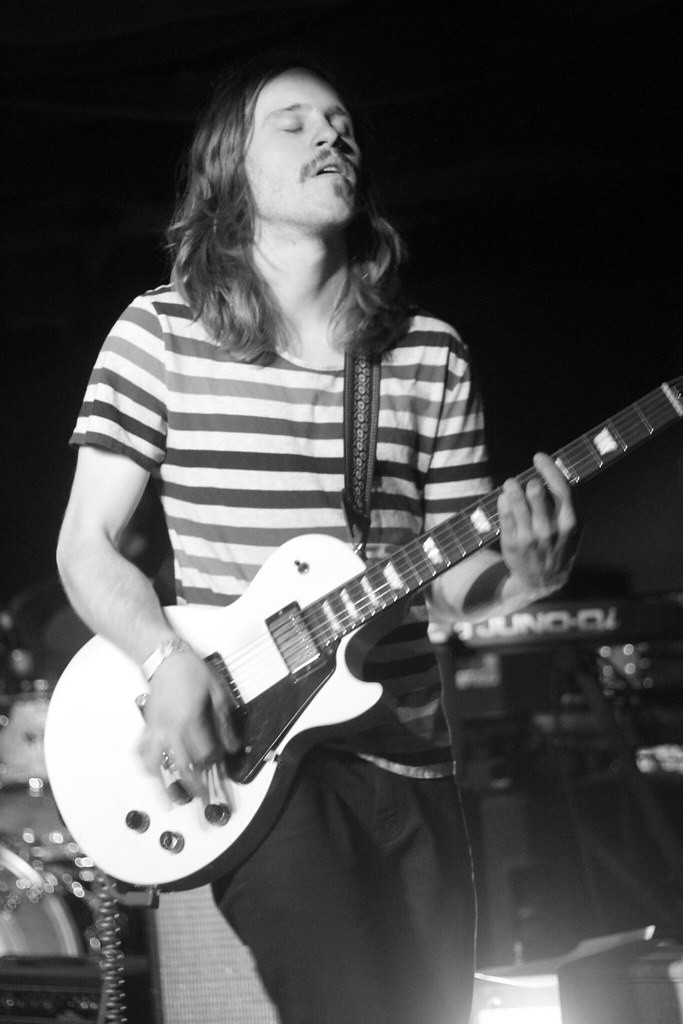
[142,639,195,682]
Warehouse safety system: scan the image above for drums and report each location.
[2,770,139,1022]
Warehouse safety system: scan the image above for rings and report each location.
[159,747,177,771]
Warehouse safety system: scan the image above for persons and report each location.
[56,62,583,1024]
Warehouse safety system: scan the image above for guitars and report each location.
[43,373,683,895]
